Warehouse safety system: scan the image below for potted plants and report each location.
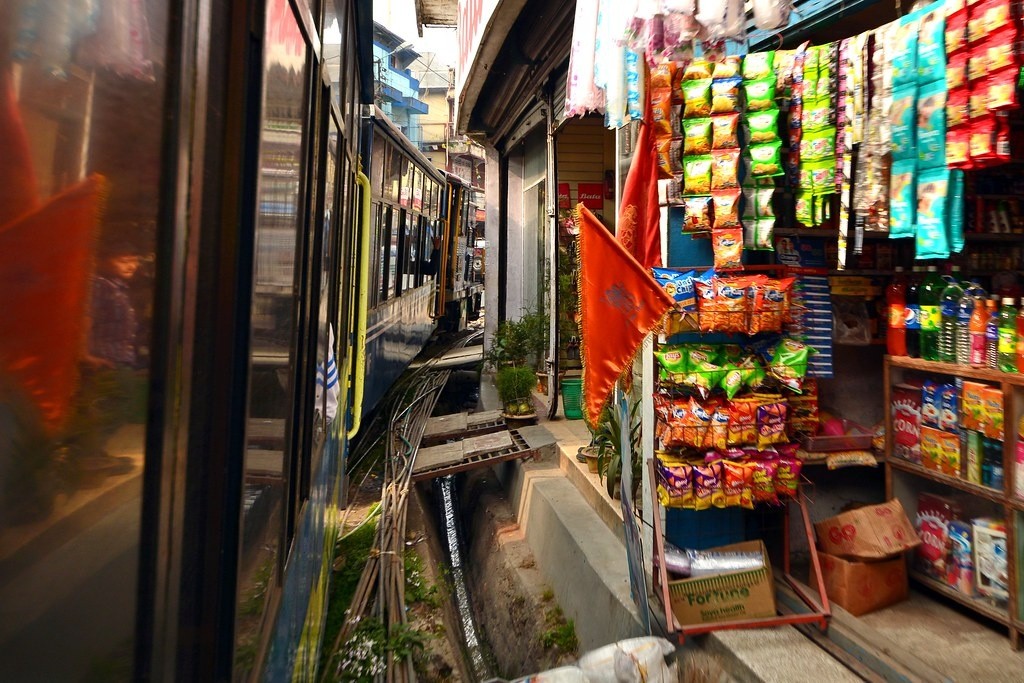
[478,244,641,502]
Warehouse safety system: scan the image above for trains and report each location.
[0,0,483,682]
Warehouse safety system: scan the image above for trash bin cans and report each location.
[561,379,583,420]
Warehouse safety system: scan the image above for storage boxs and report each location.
[667,540,777,627]
[809,375,1024,617]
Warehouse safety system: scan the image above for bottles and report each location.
[904,266,924,358]
[938,265,1024,375]
[886,265,910,357]
[919,266,947,360]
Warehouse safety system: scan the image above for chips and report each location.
[670,360,799,387]
[744,66,779,174]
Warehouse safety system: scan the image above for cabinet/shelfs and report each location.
[645,266,833,645]
[882,355,1024,649]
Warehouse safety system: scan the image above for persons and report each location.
[68,239,148,482]
[424,237,440,274]
